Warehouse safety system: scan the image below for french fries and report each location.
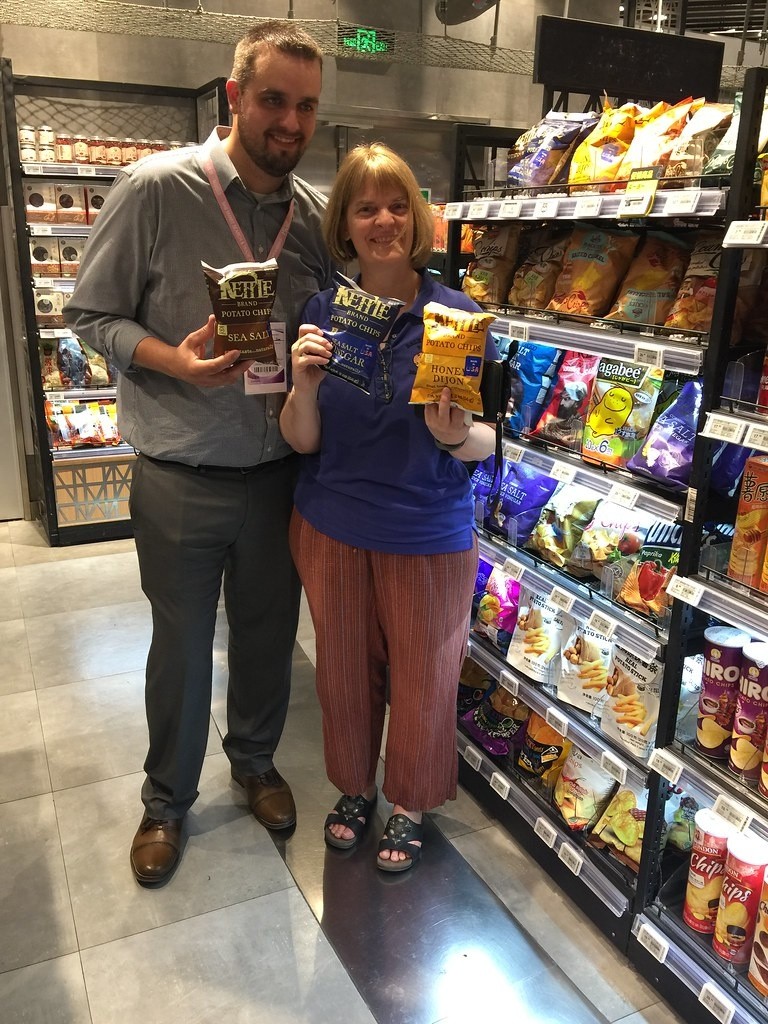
[524,626,653,736]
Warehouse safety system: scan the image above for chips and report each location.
[695,709,768,791]
[554,772,645,848]
[686,874,750,944]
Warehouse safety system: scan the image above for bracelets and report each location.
[435,438,467,451]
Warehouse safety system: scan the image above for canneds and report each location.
[17,125,200,167]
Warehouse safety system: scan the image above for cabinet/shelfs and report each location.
[0,57,236,547]
[445,67,768,1024]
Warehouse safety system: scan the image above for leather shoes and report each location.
[131,810,182,882]
[231,766,297,829]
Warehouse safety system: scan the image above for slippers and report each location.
[376,814,426,872]
[324,783,379,849]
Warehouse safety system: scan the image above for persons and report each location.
[280,145,502,872]
[63,20,329,885]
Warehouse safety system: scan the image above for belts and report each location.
[151,454,294,472]
